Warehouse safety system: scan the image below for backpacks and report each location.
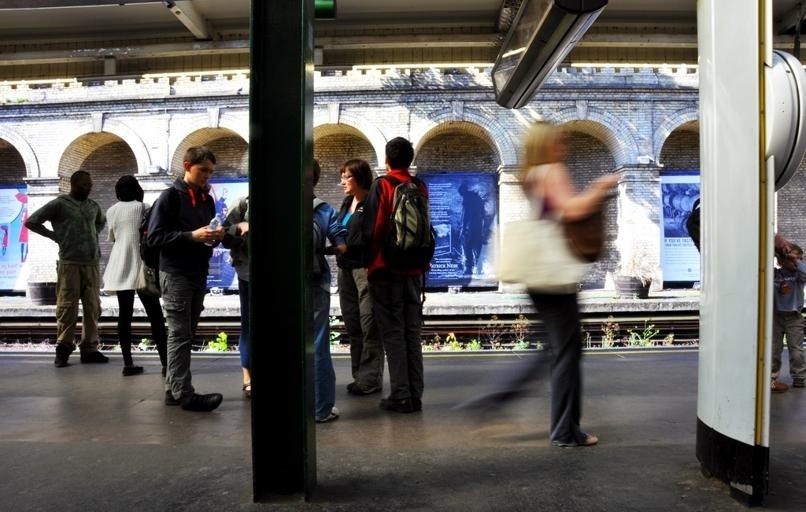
[312,196,330,289]
[684,195,699,254]
[135,185,181,272]
[372,174,436,274]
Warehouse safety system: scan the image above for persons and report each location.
[145,144,225,412]
[771,236,792,394]
[218,194,252,401]
[19,207,28,264]
[313,158,347,423]
[209,185,235,249]
[514,122,623,448]
[456,179,487,276]
[332,158,384,396]
[100,174,169,377]
[354,136,431,413]
[25,170,109,368]
[772,242,806,388]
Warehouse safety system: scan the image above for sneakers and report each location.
[345,380,383,396]
[80,350,109,363]
[314,406,340,423]
[792,377,806,387]
[178,391,223,412]
[164,383,196,406]
[771,377,790,392]
[122,364,145,377]
[54,351,72,368]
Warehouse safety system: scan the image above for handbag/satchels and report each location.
[141,259,161,299]
[490,162,606,295]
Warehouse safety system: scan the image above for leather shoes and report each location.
[379,394,423,414]
[241,381,251,399]
[551,430,598,448]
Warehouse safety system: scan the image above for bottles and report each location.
[203,212,224,248]
